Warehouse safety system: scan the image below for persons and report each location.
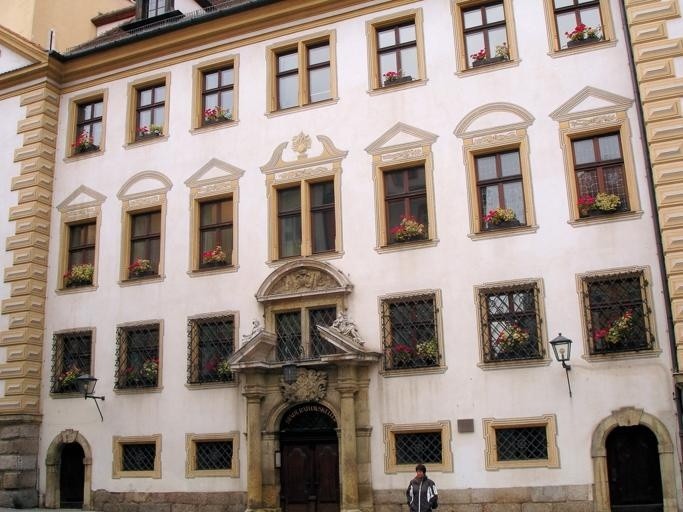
[405,464,439,512]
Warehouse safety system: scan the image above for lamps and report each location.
[78,368,105,400]
[549,332,573,371]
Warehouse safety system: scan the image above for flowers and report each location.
[200,245,227,264]
[578,192,623,212]
[203,105,231,120]
[469,46,487,62]
[593,305,634,346]
[382,69,402,80]
[390,215,426,243]
[71,127,94,148]
[564,22,598,41]
[495,322,533,354]
[483,208,518,226]
[390,337,438,362]
[63,261,93,284]
[127,256,153,276]
[136,123,162,134]
[59,368,76,384]
